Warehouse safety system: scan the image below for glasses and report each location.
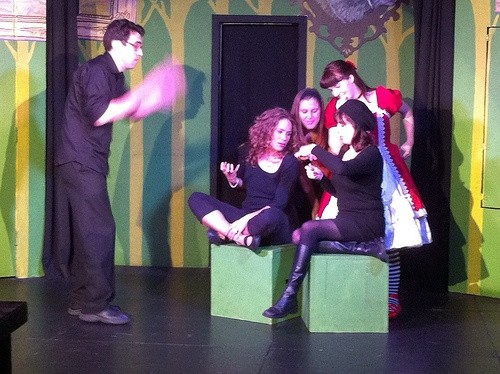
[126,41,142,51]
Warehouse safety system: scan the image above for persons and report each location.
[53,19,186,325]
[262,99,385,320]
[188,107,300,252]
[288,88,329,221]
[304,59,433,319]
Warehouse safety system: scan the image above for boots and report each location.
[263,244,313,319]
[319,237,387,261]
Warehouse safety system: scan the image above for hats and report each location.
[339,99,378,131]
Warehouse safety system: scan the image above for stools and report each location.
[209,239,304,327]
[299,251,390,334]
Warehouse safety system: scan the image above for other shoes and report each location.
[389,294,402,319]
[207,229,234,244]
[244,234,261,251]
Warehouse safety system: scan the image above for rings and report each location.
[227,172,233,175]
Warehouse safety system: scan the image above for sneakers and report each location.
[79,305,132,324]
[68,306,82,316]
